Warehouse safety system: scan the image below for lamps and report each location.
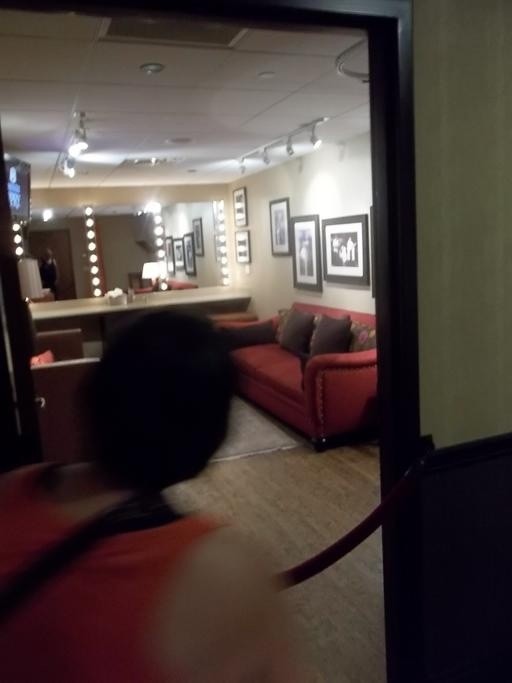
[237,117,329,173]
[84,204,103,296]
[58,111,90,179]
[11,221,25,258]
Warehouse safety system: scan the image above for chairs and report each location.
[30,327,101,465]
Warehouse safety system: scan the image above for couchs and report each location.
[216,300,377,451]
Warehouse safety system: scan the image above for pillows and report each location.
[311,314,353,356]
[279,306,313,350]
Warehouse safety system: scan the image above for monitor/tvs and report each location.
[3,154,31,220]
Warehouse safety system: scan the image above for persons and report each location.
[0,310,300,681]
[297,229,358,278]
[38,249,62,300]
[275,211,286,246]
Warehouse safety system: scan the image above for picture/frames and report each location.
[234,230,250,263]
[289,215,321,295]
[165,218,205,277]
[233,187,247,228]
[320,213,368,286]
[267,199,292,258]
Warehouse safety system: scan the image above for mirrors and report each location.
[15,185,231,304]
